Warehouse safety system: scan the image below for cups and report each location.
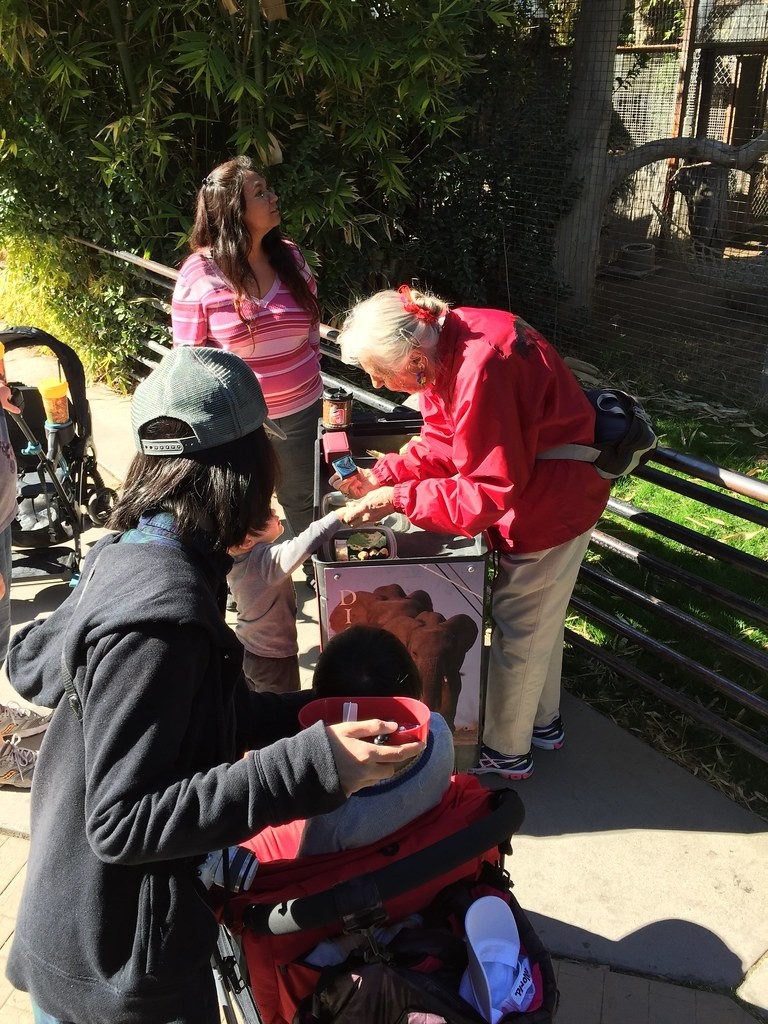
[323,388,353,431]
[38,378,68,424]
[0,342,7,384]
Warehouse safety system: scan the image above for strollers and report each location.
[207,766,560,1024]
[0,322,122,590]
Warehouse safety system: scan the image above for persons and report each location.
[0,381,54,793]
[0,351,427,1024]
[223,625,533,1024]
[172,156,321,592]
[329,289,609,781]
[225,507,349,696]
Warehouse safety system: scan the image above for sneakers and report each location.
[226,586,237,610]
[531,714,565,751]
[0,700,56,740]
[306,575,315,590]
[0,733,39,788]
[467,740,534,780]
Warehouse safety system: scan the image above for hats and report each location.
[459,896,536,1024]
[129,343,287,457]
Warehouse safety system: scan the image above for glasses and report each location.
[254,186,275,201]
